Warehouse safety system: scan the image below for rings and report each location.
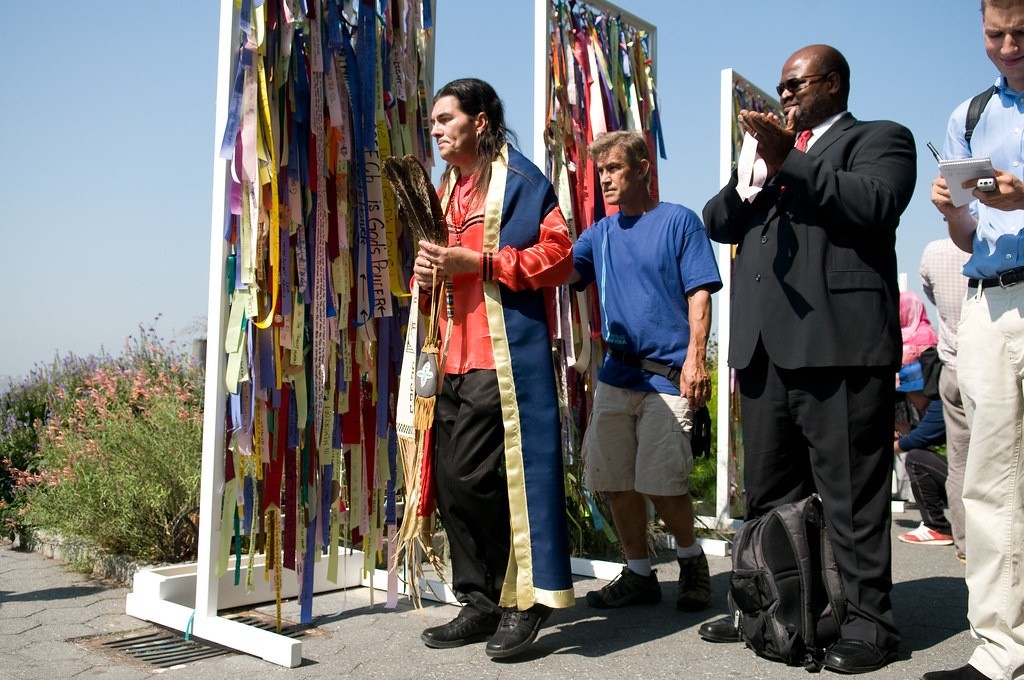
[752,132,758,137]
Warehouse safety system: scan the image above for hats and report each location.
[895,361,923,392]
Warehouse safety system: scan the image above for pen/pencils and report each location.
[926,141,944,164]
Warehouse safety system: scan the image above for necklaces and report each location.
[450,174,479,248]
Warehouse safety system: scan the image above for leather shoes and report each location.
[823,639,892,672]
[919,664,989,680]
[699,615,741,644]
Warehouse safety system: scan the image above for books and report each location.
[936,157,996,208]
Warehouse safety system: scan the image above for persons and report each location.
[920,0,1024,680]
[699,45,911,675]
[919,233,979,566]
[894,359,960,548]
[413,77,575,660]
[559,127,721,620]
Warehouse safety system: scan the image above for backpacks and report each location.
[727,494,846,674]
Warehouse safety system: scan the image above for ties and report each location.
[780,129,813,192]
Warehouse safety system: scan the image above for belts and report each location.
[967,266,1023,286]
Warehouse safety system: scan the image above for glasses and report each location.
[776,74,826,96]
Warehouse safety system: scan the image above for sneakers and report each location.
[677,545,713,614]
[587,567,662,607]
[421,603,553,659]
[898,520,953,546]
